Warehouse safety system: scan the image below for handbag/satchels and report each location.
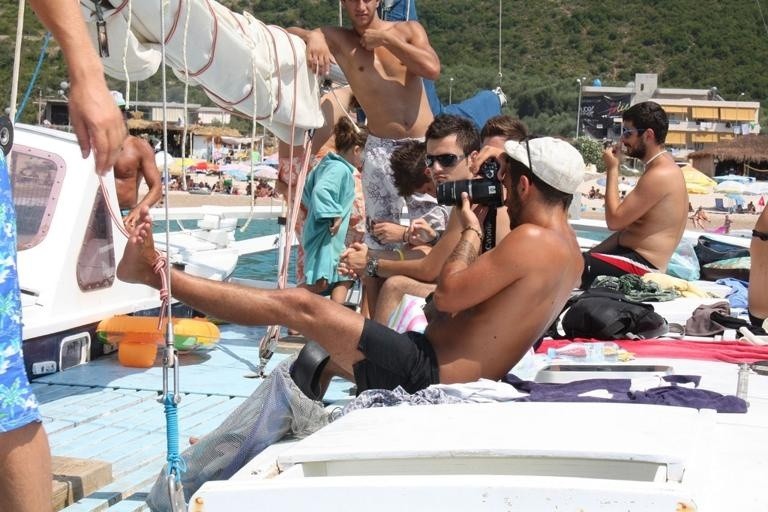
[562,287,670,340]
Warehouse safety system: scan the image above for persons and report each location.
[285,0,441,315]
[689,195,765,232]
[0,0,126,511]
[277,84,368,333]
[586,175,628,199]
[114,134,585,395]
[107,90,162,233]
[582,100,688,293]
[338,112,531,327]
[748,206,767,334]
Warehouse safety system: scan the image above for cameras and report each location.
[435,158,506,207]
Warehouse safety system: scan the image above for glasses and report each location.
[620,126,647,140]
[423,150,472,170]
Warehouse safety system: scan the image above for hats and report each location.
[504,135,587,196]
[111,89,127,110]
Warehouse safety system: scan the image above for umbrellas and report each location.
[155,149,279,197]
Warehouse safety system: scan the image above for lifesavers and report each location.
[96,314,220,368]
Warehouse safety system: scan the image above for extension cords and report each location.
[32,361,57,375]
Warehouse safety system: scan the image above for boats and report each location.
[6,118,243,385]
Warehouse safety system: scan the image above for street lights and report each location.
[448,77,454,105]
[576,77,586,138]
